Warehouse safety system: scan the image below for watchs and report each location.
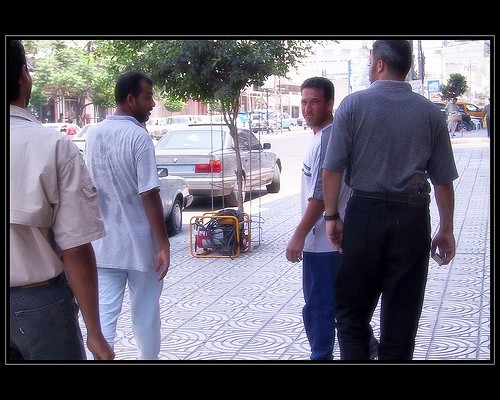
[323,210,341,221]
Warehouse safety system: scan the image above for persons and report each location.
[482,99,490,139]
[286,76,381,362]
[10,39,117,361]
[84,72,171,361]
[445,98,460,137]
[322,40,461,361]
[59,113,77,136]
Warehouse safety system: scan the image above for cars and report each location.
[145,114,246,133]
[69,123,95,152]
[40,123,81,136]
[251,112,298,132]
[154,124,283,206]
[158,166,195,237]
[433,102,470,132]
[467,119,481,131]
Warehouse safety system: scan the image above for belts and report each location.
[350,188,431,206]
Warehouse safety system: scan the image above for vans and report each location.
[442,102,483,117]
